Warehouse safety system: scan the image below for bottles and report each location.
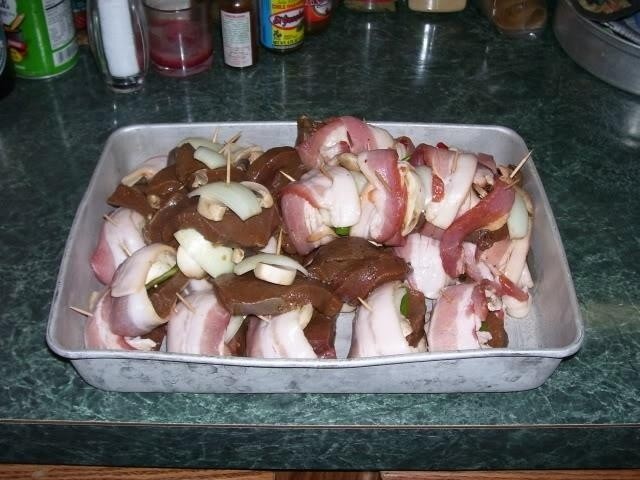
[86,0,332,96]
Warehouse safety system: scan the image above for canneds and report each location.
[0,0,82,81]
[255,0,307,55]
